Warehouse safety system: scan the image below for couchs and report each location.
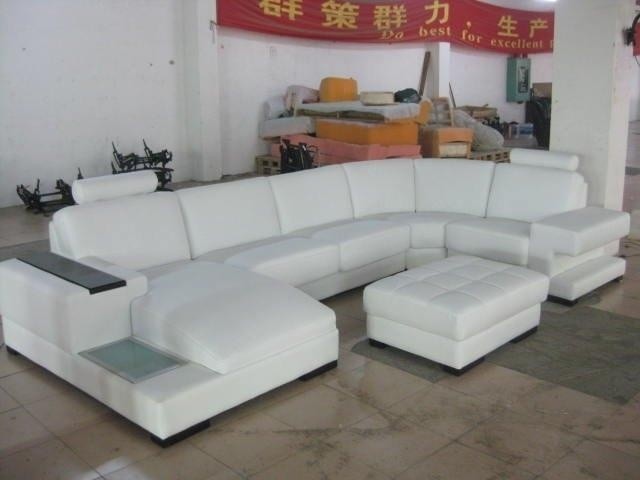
[0,146,630,443]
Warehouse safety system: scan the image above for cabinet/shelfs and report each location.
[507,57,531,103]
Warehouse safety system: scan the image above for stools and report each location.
[361,254,551,373]
